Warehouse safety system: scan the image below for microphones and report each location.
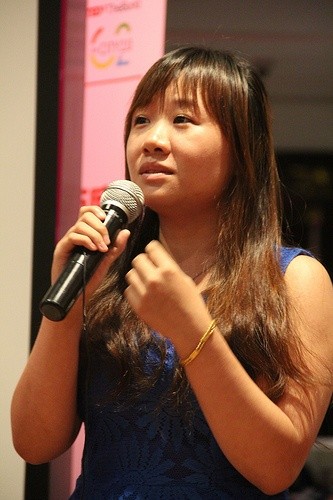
[40,178,146,323]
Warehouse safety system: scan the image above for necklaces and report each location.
[190,259,220,281]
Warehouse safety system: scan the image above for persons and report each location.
[9,45,333,499]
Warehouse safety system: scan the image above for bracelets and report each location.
[178,318,217,367]
